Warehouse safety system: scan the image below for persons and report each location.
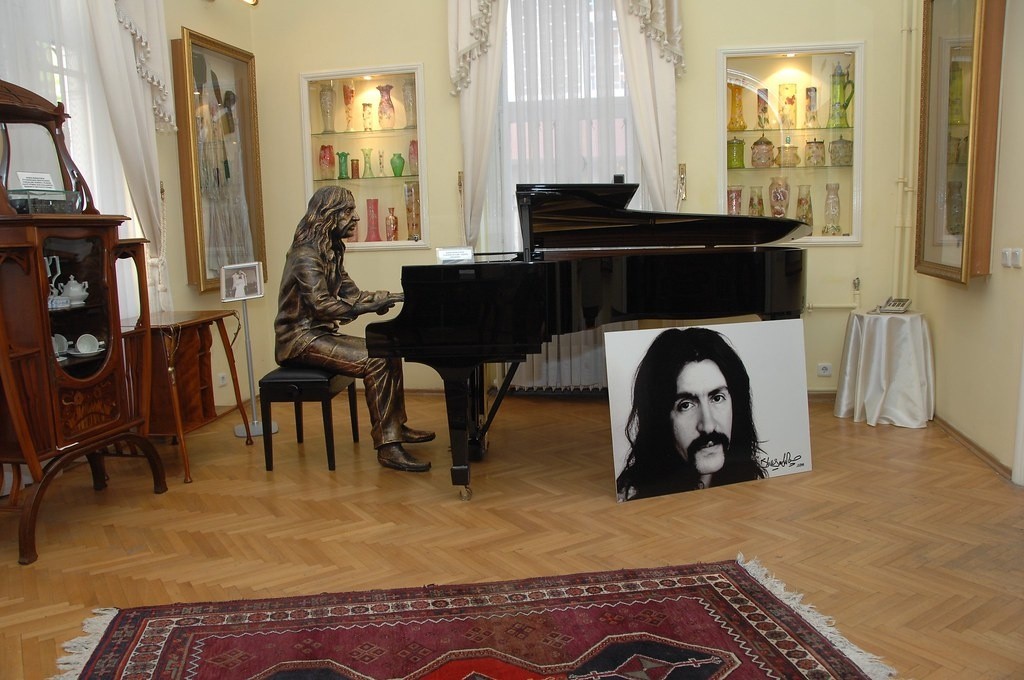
[274,185,437,472]
[616,327,770,501]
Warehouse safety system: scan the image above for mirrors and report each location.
[0,80,99,214]
[916,1,1007,287]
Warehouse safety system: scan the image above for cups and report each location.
[47,296,70,309]
[50,334,74,357]
[75,334,105,353]
[777,83,797,130]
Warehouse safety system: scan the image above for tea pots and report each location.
[58,274,89,306]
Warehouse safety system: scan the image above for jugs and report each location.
[948,62,964,125]
[826,63,855,128]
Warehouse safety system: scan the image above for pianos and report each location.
[361,171,813,502]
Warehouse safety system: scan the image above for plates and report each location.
[70,348,107,357]
[56,349,70,361]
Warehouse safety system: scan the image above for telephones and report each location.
[879,296,912,314]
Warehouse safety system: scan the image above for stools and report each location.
[257,366,359,470]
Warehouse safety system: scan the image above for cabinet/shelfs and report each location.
[299,62,430,251]
[0,209,167,567]
[717,41,866,249]
[932,32,971,248]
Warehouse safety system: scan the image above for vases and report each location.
[726,73,854,236]
[317,78,420,243]
[948,59,966,235]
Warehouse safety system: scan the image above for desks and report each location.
[119,310,255,483]
[833,310,937,428]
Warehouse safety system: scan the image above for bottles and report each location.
[727,133,854,236]
[318,141,420,180]
[946,133,970,236]
[726,78,772,131]
[345,181,422,242]
[320,80,417,133]
[802,87,818,129]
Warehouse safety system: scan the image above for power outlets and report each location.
[817,363,834,378]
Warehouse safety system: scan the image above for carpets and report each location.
[53,557,904,680]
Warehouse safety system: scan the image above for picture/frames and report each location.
[168,25,270,294]
